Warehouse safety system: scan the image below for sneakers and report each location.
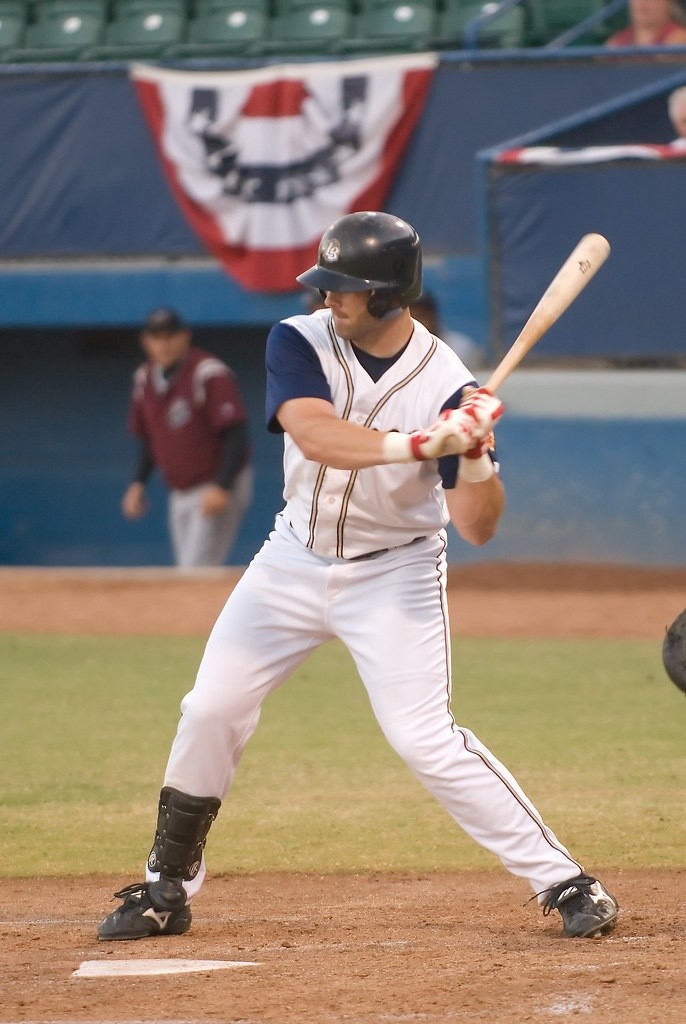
[543,873,620,939]
[98,881,193,940]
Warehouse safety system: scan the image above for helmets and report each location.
[295,211,423,320]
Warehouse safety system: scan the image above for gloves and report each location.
[383,383,506,484]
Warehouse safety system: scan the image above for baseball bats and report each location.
[442,232,613,454]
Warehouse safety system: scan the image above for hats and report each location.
[138,306,185,337]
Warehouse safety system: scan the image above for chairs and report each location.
[0,1,630,68]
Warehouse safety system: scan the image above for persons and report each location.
[121,310,254,567]
[592,0,686,63]
[88,208,621,944]
